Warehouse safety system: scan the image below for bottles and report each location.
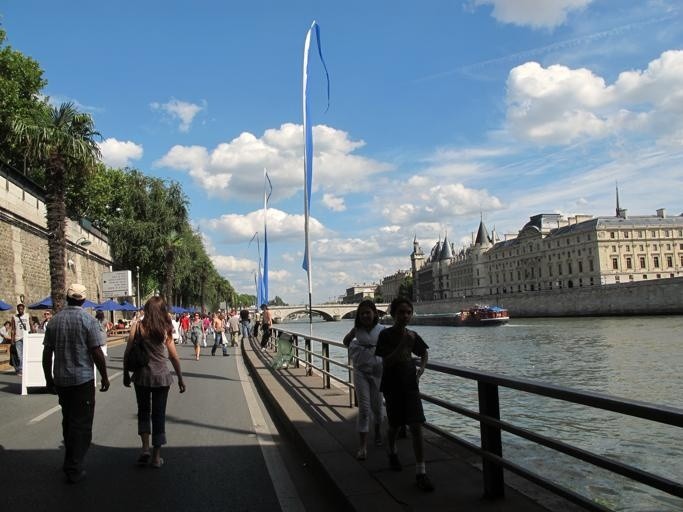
[67,283,88,300]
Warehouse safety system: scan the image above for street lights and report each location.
[126,333,149,372]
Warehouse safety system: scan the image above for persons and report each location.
[0,304,52,376]
[375,298,436,492]
[90,308,271,360]
[342,299,387,461]
[42,284,110,485]
[123,296,185,468]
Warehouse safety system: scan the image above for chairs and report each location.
[416,472,436,492]
[63,464,87,483]
[135,452,150,466]
[357,445,369,461]
[211,351,215,356]
[387,452,403,470]
[223,353,230,356]
[151,457,165,468]
[375,435,385,449]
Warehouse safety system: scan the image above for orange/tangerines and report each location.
[269,331,297,374]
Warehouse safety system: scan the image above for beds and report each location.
[27,292,139,325]
[0,300,13,311]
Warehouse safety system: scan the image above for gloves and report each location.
[378,301,511,328]
